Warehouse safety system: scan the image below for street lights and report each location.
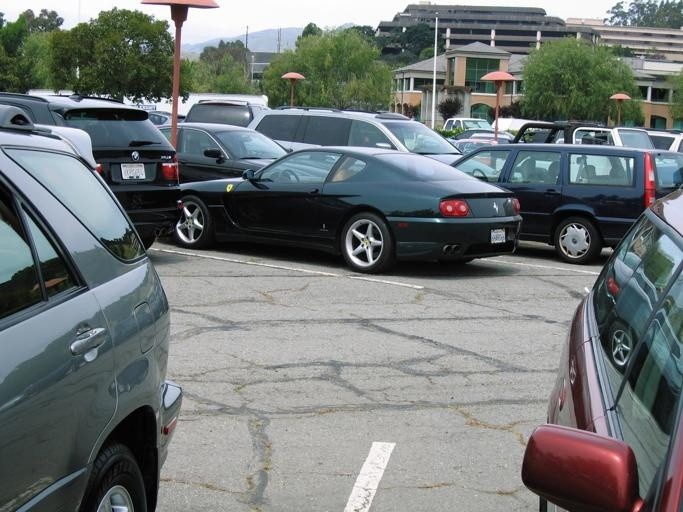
[280,71,304,109]
[479,71,517,144]
[139,0,220,149]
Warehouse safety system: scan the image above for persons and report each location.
[521,158,538,179]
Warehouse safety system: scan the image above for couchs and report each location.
[577,165,624,184]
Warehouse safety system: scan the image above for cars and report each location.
[516,182,683,511]
[593,244,683,434]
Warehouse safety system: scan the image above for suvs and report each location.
[0,105,184,509]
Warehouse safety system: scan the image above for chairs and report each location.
[543,161,559,183]
[520,158,536,182]
